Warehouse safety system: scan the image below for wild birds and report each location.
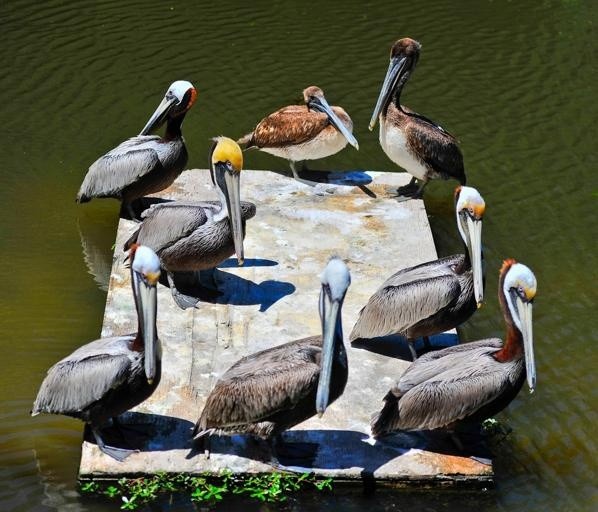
[27,243,163,464]
[188,254,352,466]
[72,78,198,223]
[372,258,539,467]
[230,84,361,189]
[364,36,468,204]
[348,185,487,362]
[121,134,258,312]
